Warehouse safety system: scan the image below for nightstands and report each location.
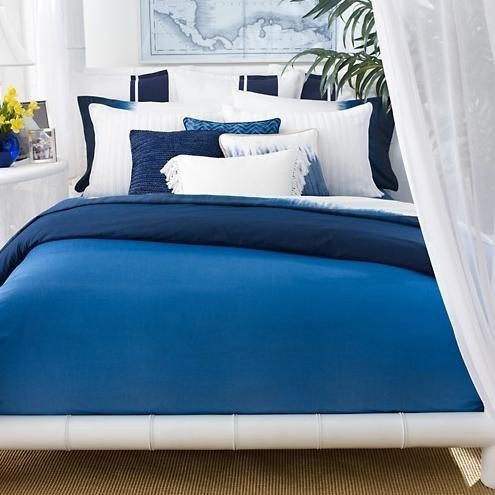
[0,160,70,250]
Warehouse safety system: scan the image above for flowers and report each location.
[0,88,40,132]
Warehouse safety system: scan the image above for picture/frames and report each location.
[28,126,58,163]
[139,0,340,68]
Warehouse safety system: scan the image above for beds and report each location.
[1,70,483,413]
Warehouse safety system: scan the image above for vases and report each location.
[1,128,20,167]
